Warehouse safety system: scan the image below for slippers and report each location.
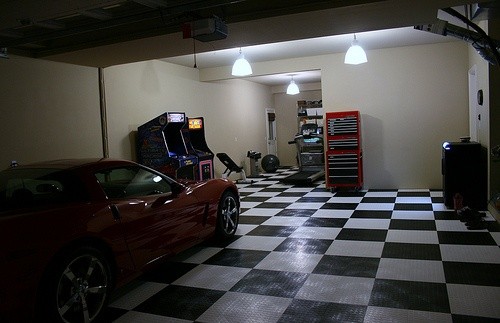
[459,209,486,222]
[466,221,495,230]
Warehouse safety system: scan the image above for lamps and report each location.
[286,75,300,95]
[231,47,253,77]
[344,33,368,65]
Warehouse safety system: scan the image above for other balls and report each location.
[261,154,280,172]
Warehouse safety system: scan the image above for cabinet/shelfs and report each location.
[296,101,324,136]
[326,111,363,192]
[441,141,489,212]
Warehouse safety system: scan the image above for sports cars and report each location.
[0,158,241,323]
